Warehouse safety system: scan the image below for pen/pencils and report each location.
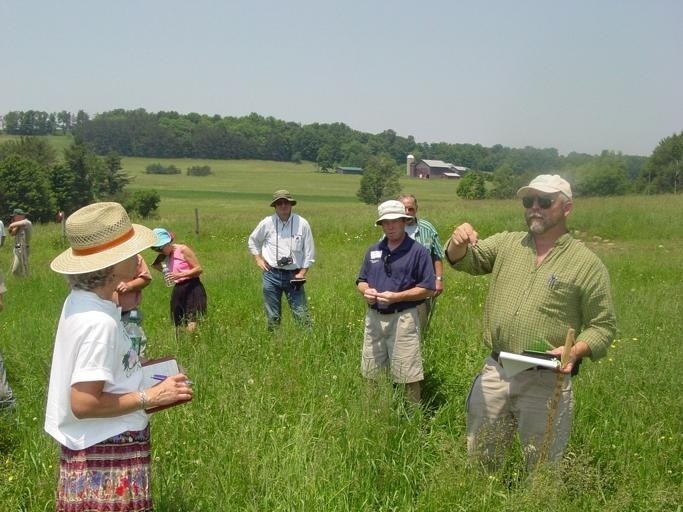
[149,375,195,385]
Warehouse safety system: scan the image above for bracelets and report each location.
[436,276,444,282]
[138,390,148,410]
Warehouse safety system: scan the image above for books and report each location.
[141,356,192,413]
[498,349,561,378]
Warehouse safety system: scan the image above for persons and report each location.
[355,200,436,403]
[149,229,207,335]
[44,201,192,512]
[0,220,6,247]
[0,262,15,411]
[8,209,32,278]
[444,174,617,472]
[112,254,152,359]
[55,209,64,223]
[247,190,316,332]
[378,193,444,328]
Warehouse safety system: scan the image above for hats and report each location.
[49,201,160,275]
[269,190,295,207]
[9,209,29,216]
[149,228,171,247]
[516,174,571,202]
[375,200,414,225]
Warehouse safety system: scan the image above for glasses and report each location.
[277,199,289,205]
[522,196,554,209]
[405,208,413,212]
[153,246,164,252]
[383,253,391,273]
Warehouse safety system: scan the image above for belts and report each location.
[490,351,547,370]
[271,268,299,273]
[368,304,405,315]
[15,246,29,248]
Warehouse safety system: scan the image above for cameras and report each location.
[277,256,293,267]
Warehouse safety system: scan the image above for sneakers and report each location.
[0,395,17,410]
